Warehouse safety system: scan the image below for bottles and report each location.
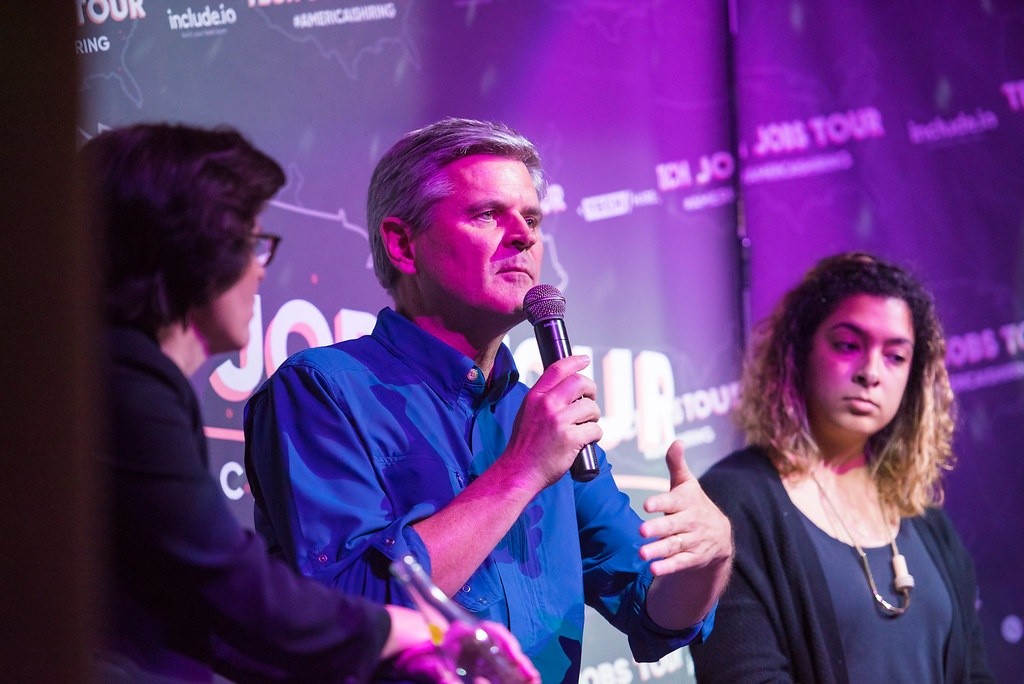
[389,554,541,684]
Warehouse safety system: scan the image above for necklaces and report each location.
[812,471,915,614]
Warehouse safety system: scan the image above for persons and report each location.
[83,124,540,684]
[243,119,734,684]
[688,253,996,684]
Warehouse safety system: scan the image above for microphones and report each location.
[523,283,599,484]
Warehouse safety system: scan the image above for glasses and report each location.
[246,233,282,267]
